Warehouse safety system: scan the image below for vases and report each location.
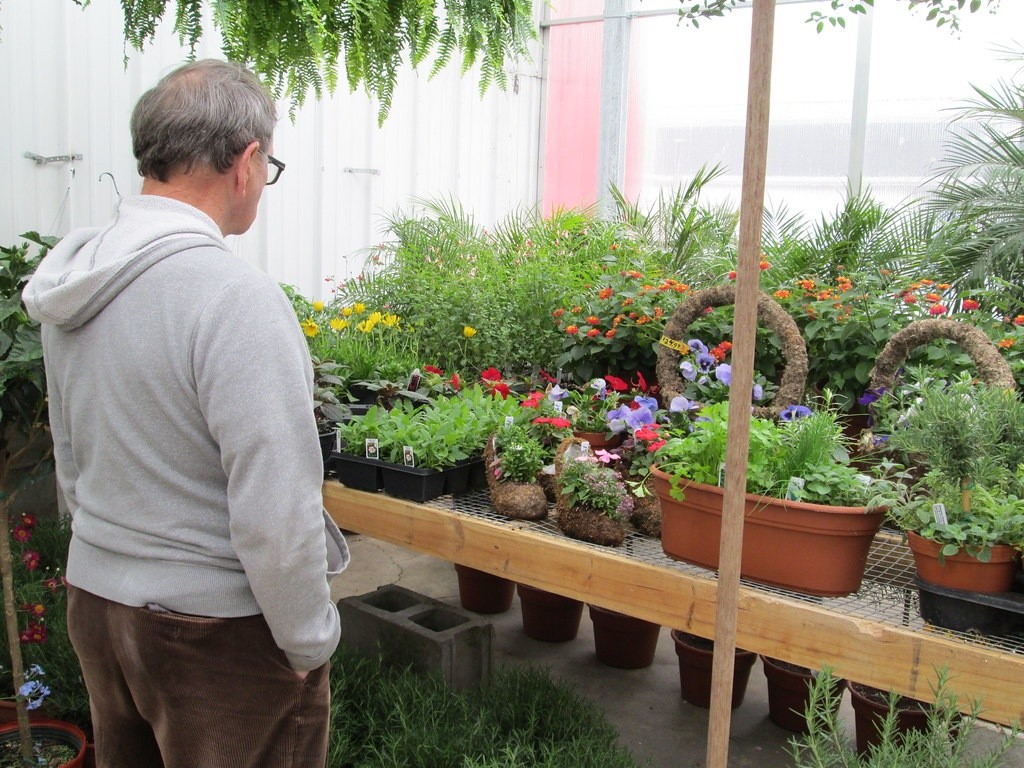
[454,565,964,760]
[0,721,87,768]
[810,380,876,455]
[649,464,891,597]
[0,700,36,722]
[321,430,336,460]
[83,742,96,768]
[580,431,622,468]
[331,448,491,503]
[906,532,1016,591]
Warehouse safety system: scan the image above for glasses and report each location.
[232,139,286,185]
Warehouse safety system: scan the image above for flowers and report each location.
[0,511,92,737]
[277,159,1024,565]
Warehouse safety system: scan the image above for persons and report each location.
[39,60,351,768]
